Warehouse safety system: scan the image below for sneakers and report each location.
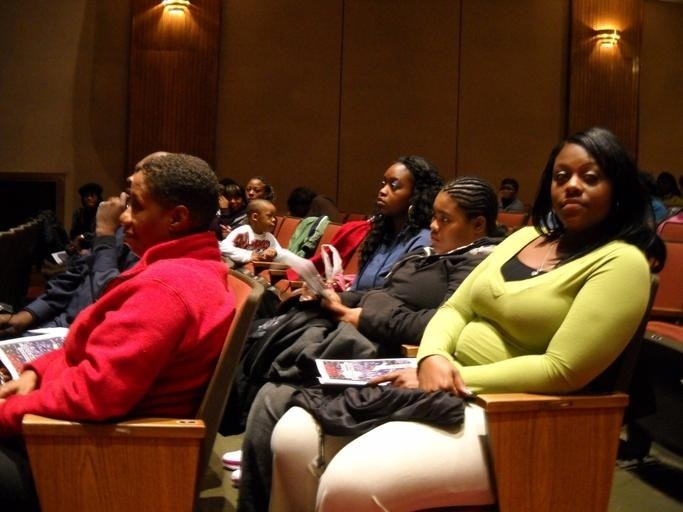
[221,449,243,471]
[229,469,241,486]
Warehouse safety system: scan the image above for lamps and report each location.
[151,0,193,17]
[589,24,624,51]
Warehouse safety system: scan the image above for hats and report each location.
[497,177,520,193]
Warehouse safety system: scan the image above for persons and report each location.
[0,148,232,512]
[273,126,668,512]
[654,173,683,220]
[217,154,528,510]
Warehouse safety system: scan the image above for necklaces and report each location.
[531,237,560,276]
